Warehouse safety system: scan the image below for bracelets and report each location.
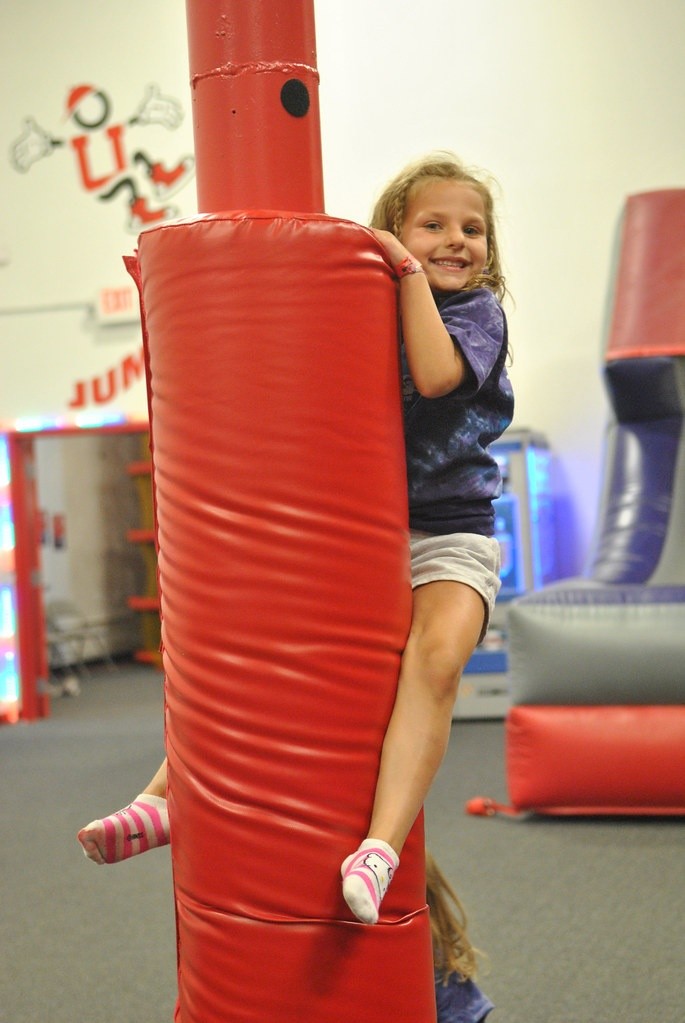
[395,254,425,279]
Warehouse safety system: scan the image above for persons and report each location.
[75,152,514,918]
[420,843,494,1023]
